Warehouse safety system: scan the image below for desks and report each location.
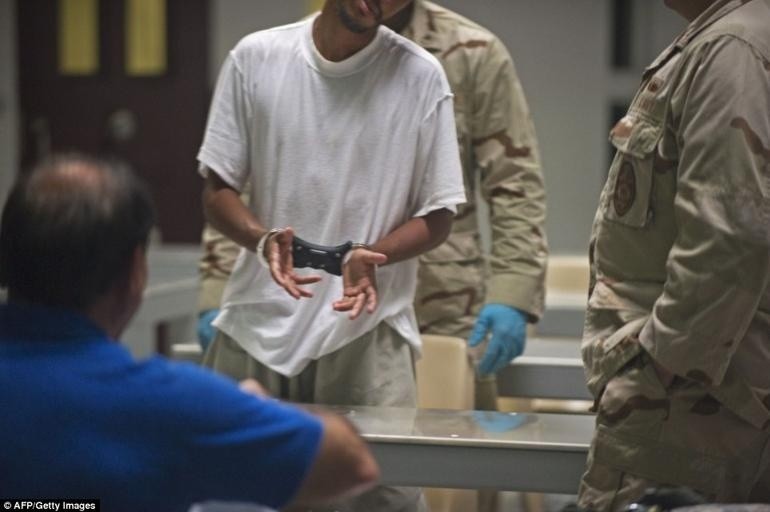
[286,291,596,511]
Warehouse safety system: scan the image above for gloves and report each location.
[195,312,220,353]
[468,304,526,376]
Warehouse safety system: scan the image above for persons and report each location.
[196,0,468,511]
[1,146,382,511]
[197,0,550,511]
[572,0,770,512]
